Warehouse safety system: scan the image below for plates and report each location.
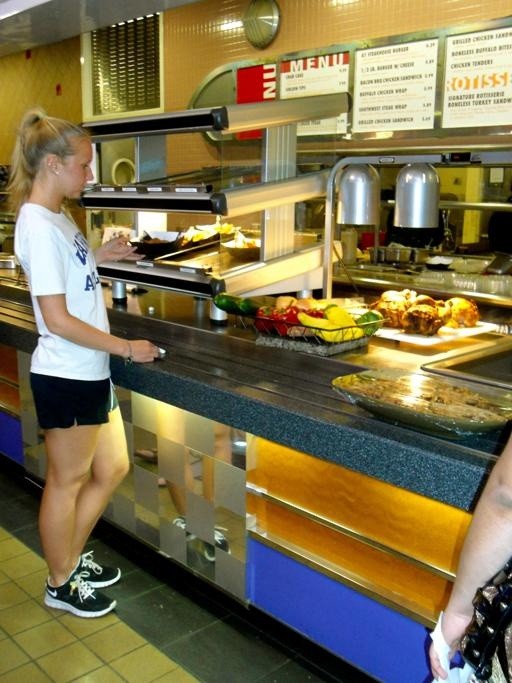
[372,321,499,347]
[219,238,260,255]
[331,368,512,439]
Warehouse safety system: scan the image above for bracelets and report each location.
[124,341,133,368]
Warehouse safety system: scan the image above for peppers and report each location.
[255,305,323,336]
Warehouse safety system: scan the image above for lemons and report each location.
[275,295,297,309]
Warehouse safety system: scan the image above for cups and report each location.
[340,232,357,265]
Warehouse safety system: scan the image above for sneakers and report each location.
[44,567,118,619]
[80,552,123,590]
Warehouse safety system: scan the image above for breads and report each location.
[369,288,481,335]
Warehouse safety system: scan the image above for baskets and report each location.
[235,316,390,356]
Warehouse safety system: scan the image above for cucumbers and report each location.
[211,289,266,318]
[298,305,381,342]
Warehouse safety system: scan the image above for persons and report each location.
[385,203,445,249]
[488,179,512,253]
[426,431,512,683]
[5,108,161,618]
[133,400,232,562]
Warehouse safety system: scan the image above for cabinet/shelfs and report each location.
[71,91,354,324]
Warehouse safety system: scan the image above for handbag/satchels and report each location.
[444,560,511,678]
